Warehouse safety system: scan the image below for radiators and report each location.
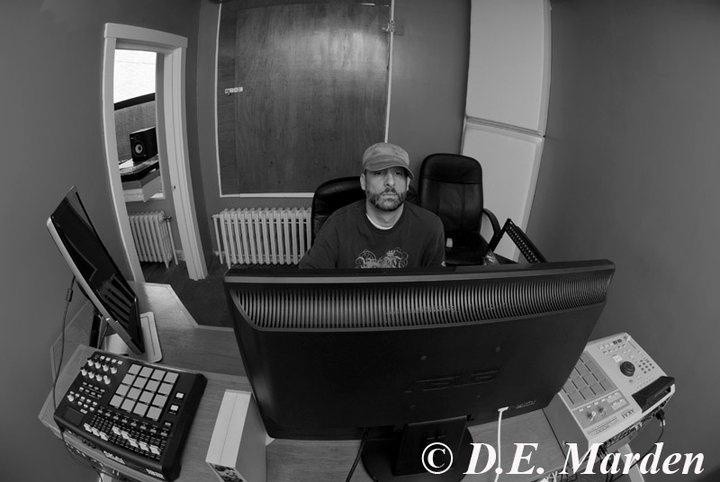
[129,210,174,267]
[210,208,311,270]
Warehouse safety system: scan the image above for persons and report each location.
[298,140,447,270]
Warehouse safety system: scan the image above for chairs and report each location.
[310,151,507,270]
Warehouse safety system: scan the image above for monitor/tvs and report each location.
[47,185,163,364]
[219,260,618,482]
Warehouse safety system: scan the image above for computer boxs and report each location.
[203,388,266,482]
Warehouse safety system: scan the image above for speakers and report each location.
[129,126,156,165]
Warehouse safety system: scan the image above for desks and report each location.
[120,158,159,203]
[36,340,579,482]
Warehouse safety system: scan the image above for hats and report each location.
[363,142,414,180]
